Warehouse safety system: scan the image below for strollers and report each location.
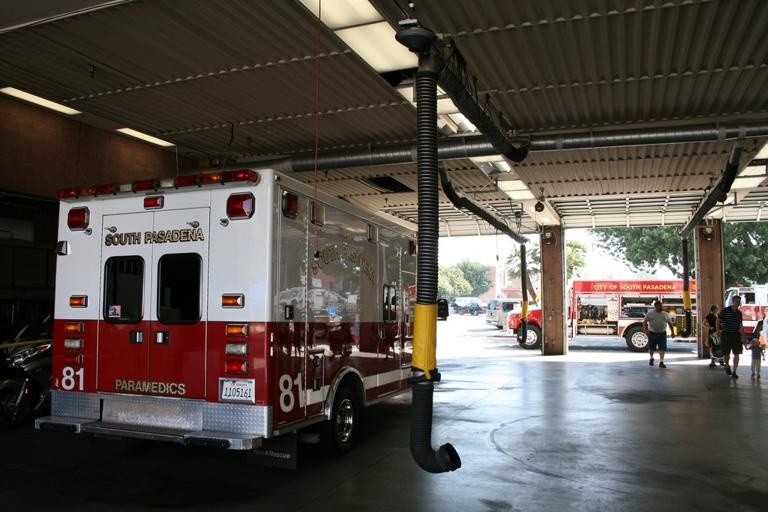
[708,326,731,369]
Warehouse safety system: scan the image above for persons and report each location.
[643,302,676,368]
[701,295,768,378]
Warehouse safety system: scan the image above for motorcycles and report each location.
[0,314,52,427]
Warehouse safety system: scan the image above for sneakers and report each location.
[718,359,724,365]
[752,373,755,377]
[757,373,760,378]
[708,363,716,368]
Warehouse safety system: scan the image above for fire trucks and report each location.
[507,277,698,353]
[33,168,449,469]
[724,281,768,338]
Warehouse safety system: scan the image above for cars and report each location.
[459,303,487,315]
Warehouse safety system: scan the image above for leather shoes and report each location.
[650,359,653,365]
[725,364,731,375]
[732,373,738,378]
[659,363,666,368]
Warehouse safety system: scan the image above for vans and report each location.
[485,299,536,329]
[451,297,482,313]
